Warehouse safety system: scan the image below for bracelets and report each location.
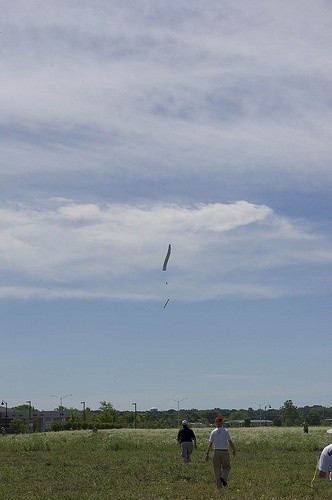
[206,453,209,454]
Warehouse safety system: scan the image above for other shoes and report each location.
[219,476,227,487]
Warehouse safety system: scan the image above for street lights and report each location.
[132,403,136,428]
[169,398,188,428]
[249,401,270,427]
[81,401,85,421]
[264,405,271,425]
[26,400,31,433]
[51,394,72,422]
[1,399,8,433]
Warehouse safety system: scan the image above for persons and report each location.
[317,429,332,478]
[206,416,236,489]
[178,420,197,463]
[303,420,309,433]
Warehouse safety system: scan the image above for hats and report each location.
[182,420,188,425]
[215,416,223,422]
